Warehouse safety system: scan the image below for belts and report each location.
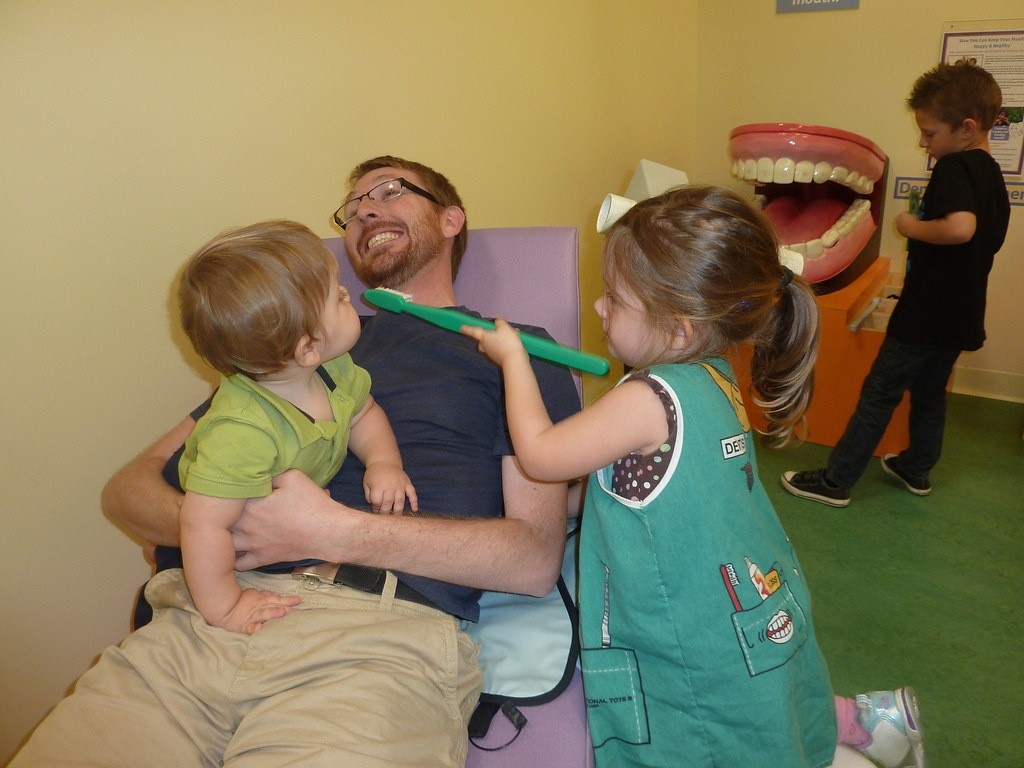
[254,561,446,611]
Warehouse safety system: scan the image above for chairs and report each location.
[316,227,596,768]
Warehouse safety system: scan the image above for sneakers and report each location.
[881,452,933,496]
[854,685,924,768]
[781,468,850,507]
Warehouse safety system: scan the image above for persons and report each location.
[130,221,420,637]
[462,186,930,767]
[774,57,1013,510]
[0,154,587,767]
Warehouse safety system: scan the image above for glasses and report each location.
[331,177,447,230]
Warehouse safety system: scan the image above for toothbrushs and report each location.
[361,286,612,378]
